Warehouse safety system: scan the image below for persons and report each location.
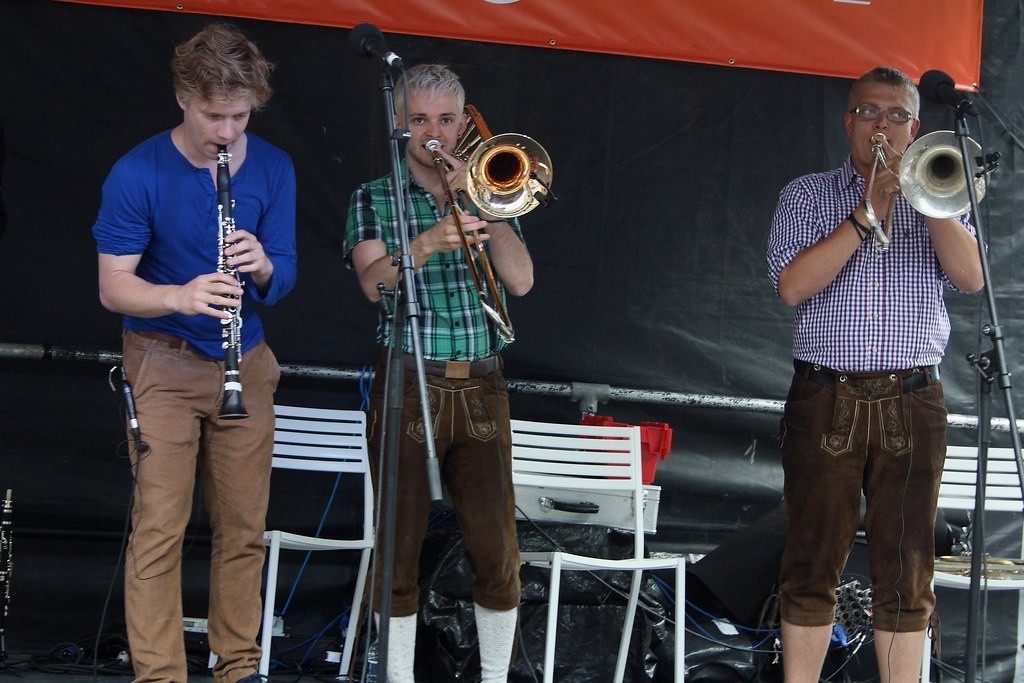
[342,65,535,683]
[764,65,987,683]
[92,24,299,683]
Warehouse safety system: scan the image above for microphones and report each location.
[120,379,141,444]
[918,68,984,118]
[346,21,405,71]
[425,140,442,164]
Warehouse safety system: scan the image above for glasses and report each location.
[850,102,917,124]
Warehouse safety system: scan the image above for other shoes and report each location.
[236,674,271,683]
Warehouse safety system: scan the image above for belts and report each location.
[793,358,941,396]
[371,343,500,379]
[131,330,205,355]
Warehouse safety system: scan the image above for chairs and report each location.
[509,419,686,683]
[920,446,1024,683]
[206,406,376,683]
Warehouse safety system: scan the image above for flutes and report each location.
[0,490,15,668]
[206,143,252,421]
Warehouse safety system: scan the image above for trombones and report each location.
[861,129,990,252]
[424,103,555,345]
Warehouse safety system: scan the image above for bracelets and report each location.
[480,219,513,226]
[845,212,872,242]
